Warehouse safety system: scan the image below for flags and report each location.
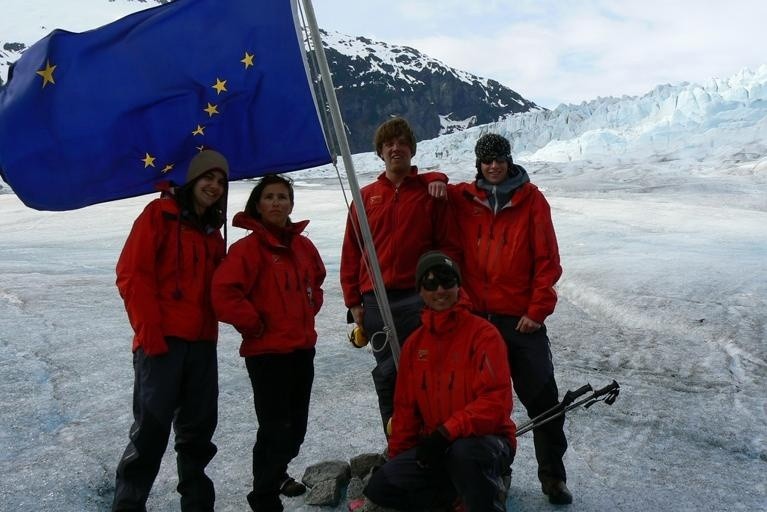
[0,1,332,210]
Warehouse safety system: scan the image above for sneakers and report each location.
[537,473,574,505]
[247,470,307,512]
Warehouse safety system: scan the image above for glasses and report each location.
[476,152,508,165]
[419,274,459,292]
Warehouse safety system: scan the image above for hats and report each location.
[412,247,464,293]
[474,133,514,168]
[183,147,232,186]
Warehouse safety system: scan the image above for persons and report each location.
[212,174,326,511]
[340,118,449,439]
[428,134,572,505]
[113,150,229,512]
[366,250,517,511]
